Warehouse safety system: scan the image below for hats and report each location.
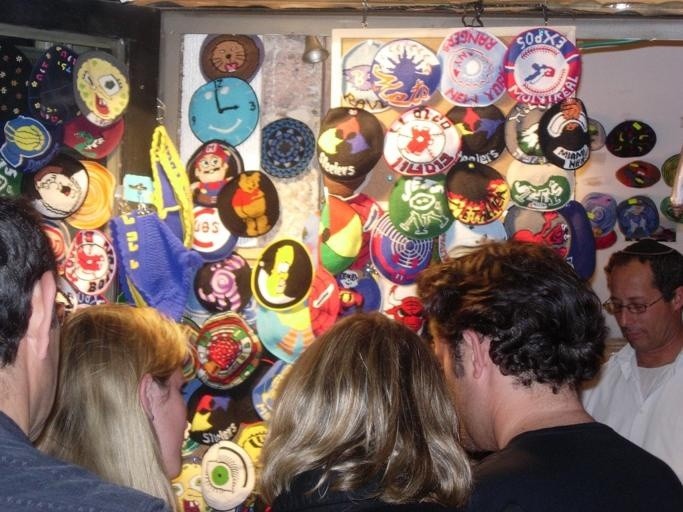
[0,27,683,511]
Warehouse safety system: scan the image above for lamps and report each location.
[303,32,330,62]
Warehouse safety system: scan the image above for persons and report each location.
[257,308,476,511]
[413,235,682,510]
[29,302,201,512]
[0,193,178,512]
[569,240,682,484]
